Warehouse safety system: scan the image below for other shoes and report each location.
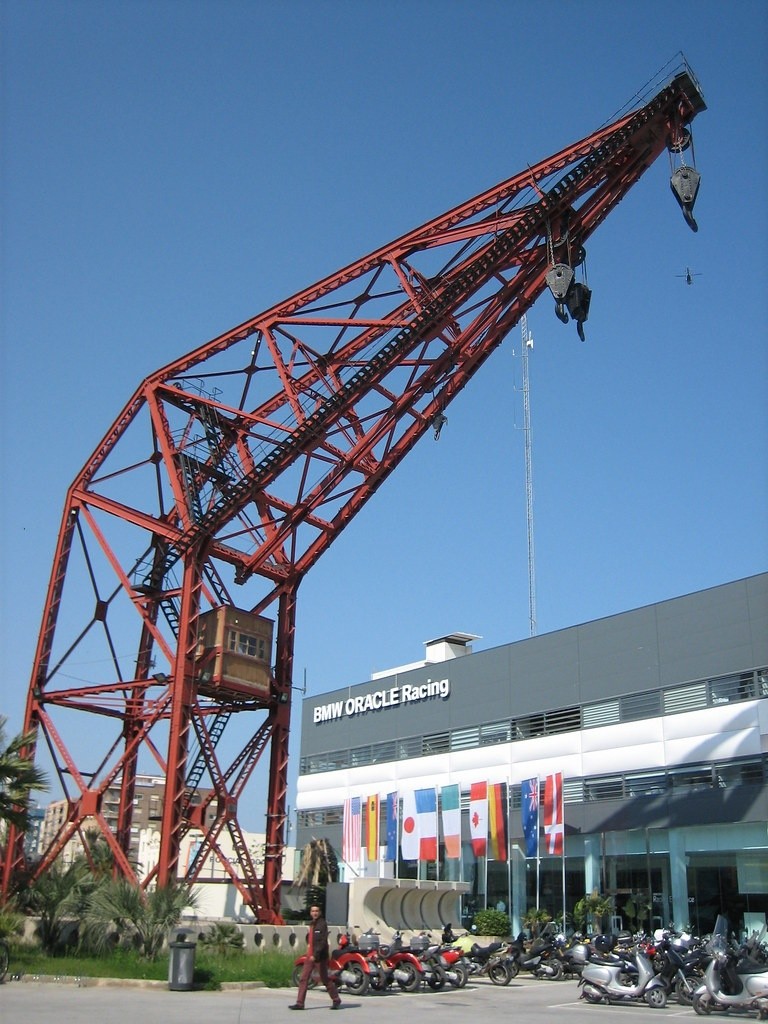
[288,1004,304,1010]
[330,1000,341,1009]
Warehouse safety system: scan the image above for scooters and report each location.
[576,944,667,1008]
[691,914,768,1019]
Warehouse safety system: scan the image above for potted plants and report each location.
[471,907,516,949]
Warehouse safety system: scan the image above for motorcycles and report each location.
[291,922,653,996]
[613,925,767,1003]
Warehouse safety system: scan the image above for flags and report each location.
[488,782,509,862]
[401,788,419,860]
[340,797,361,864]
[366,794,379,861]
[440,784,461,859]
[468,781,487,857]
[385,791,397,860]
[543,772,563,854]
[415,787,438,862]
[521,777,538,858]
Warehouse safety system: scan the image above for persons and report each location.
[287,903,343,1012]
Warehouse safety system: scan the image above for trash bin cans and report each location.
[167,941,198,991]
[408,935,431,950]
[357,933,380,950]
